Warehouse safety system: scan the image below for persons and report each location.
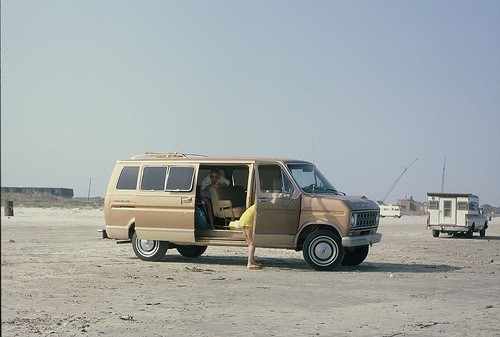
[229,205,263,270]
[200,168,231,229]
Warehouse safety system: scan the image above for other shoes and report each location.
[247,264,262,269]
[254,261,263,266]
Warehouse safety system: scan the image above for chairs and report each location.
[272,177,284,192]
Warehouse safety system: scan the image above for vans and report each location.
[379,203,402,218]
[97,151,384,272]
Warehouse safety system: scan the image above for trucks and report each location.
[425,192,491,238]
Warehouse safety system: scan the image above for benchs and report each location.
[204,184,247,229]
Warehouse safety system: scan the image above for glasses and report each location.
[210,175,219,178]
